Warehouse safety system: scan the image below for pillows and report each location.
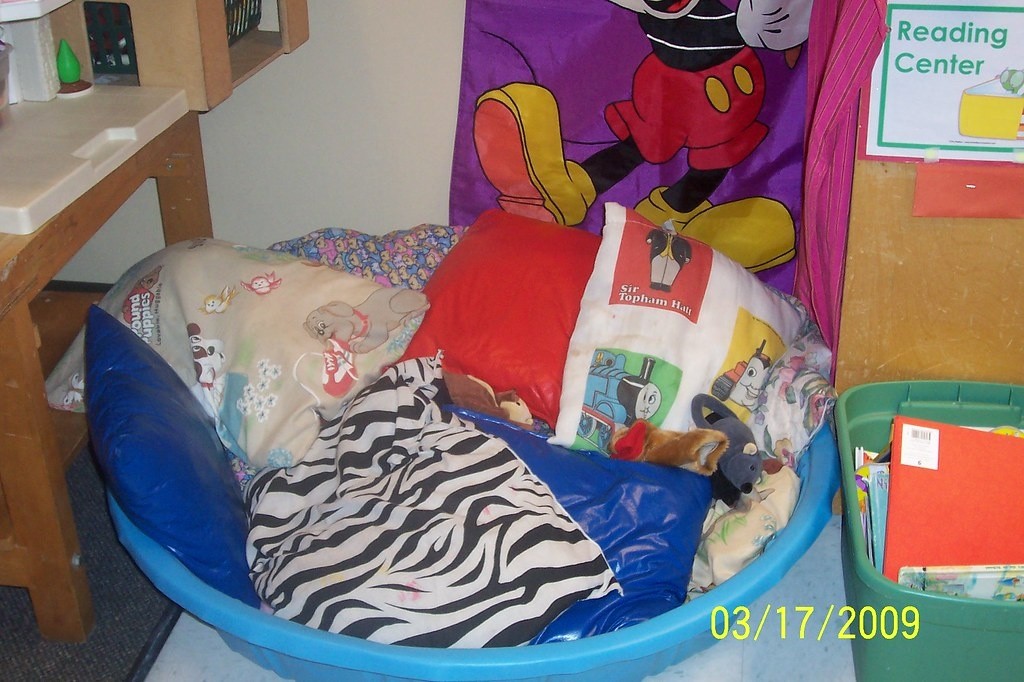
[245,349,624,649]
[547,201,800,459]
[85,302,261,610]
[428,398,712,645]
[45,237,431,467]
[383,207,603,427]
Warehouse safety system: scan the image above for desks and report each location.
[0,0,310,642]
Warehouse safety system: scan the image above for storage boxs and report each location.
[84,0,262,75]
[834,381,1024,682]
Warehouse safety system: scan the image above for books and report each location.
[851,415,1024,610]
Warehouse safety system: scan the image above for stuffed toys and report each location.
[608,393,760,504]
[440,368,536,432]
